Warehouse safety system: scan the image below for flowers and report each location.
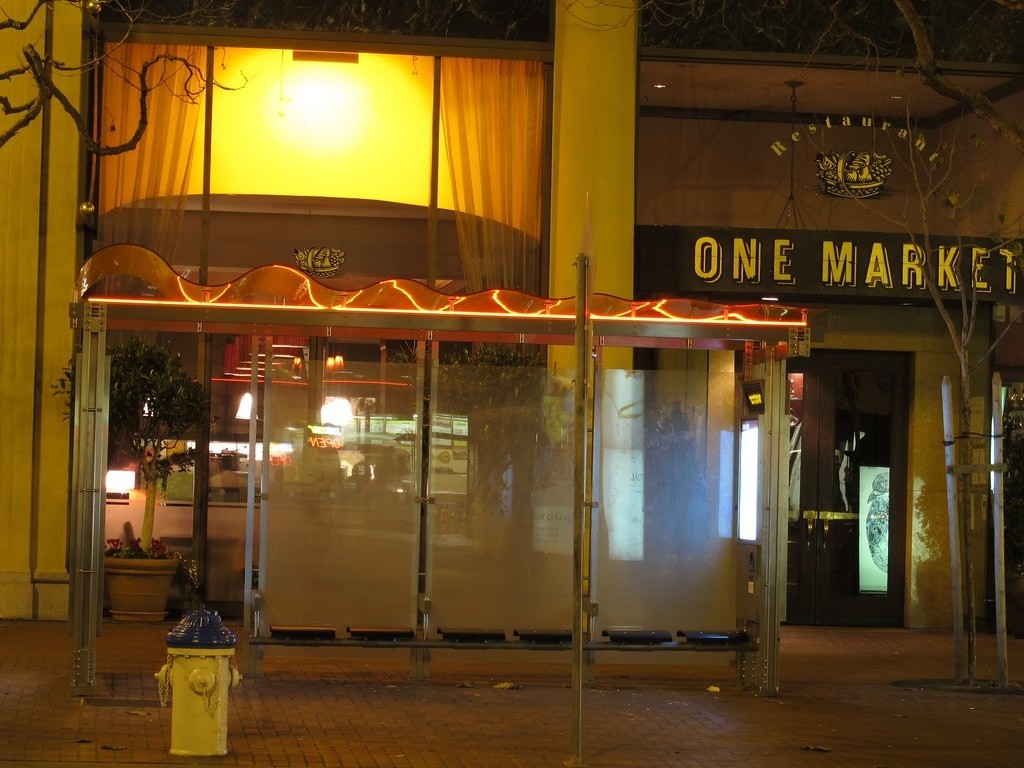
[50,333,221,559]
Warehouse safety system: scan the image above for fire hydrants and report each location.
[154,603,243,757]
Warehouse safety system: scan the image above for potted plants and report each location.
[1005,437,1024,638]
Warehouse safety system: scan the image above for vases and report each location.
[103,560,180,621]
